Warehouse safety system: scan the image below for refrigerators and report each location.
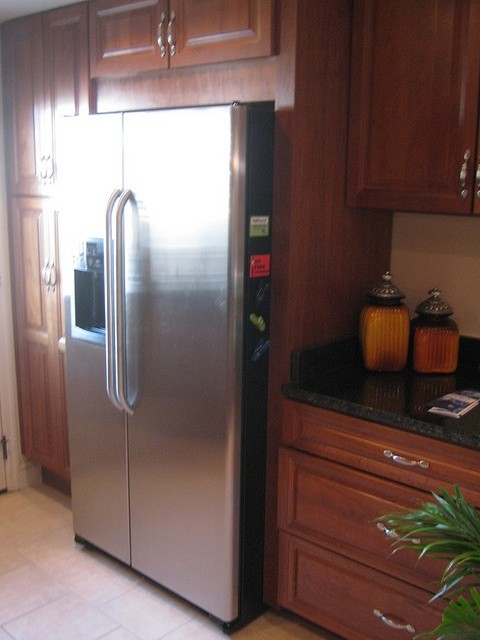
[54,98,276,633]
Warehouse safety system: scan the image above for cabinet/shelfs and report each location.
[0,1,93,196]
[7,197,73,496]
[344,0,480,216]
[274,397,480,639]
[88,1,353,81]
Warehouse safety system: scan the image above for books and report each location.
[425,389,480,419]
